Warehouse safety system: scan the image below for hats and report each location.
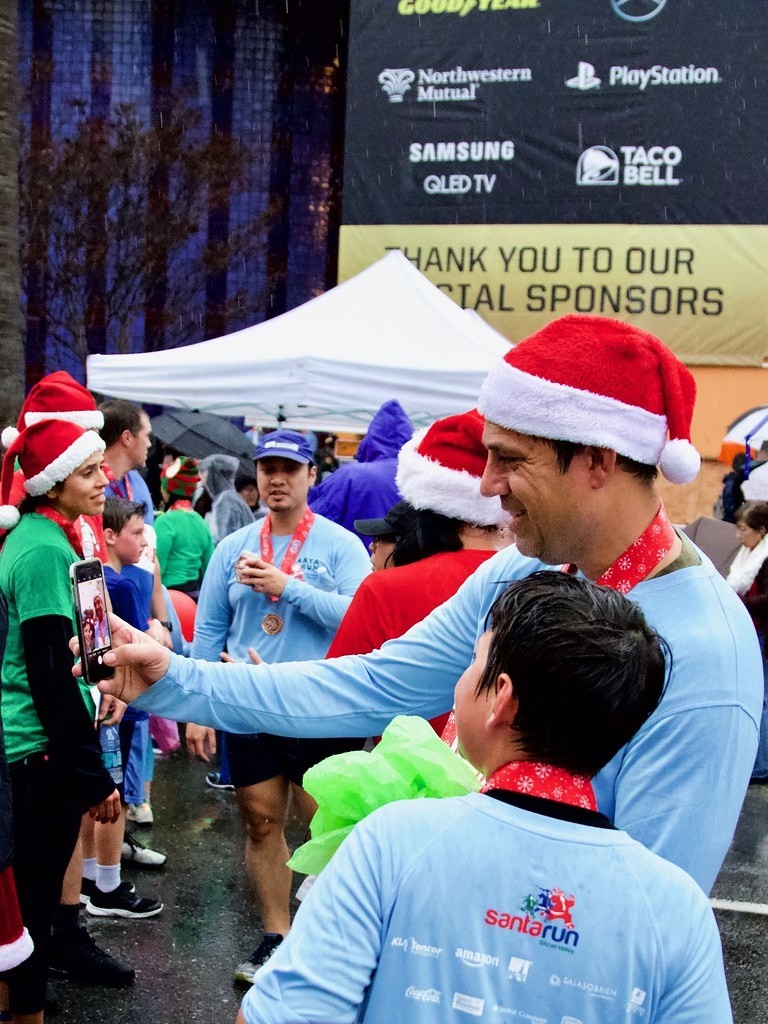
[161,456,200,496]
[1,370,104,448]
[733,454,754,469]
[478,315,701,484]
[354,500,410,537]
[253,430,315,466]
[395,408,511,528]
[0,420,106,529]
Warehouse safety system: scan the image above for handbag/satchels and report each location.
[713,494,725,520]
[147,714,180,756]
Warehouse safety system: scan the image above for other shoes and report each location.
[126,802,153,823]
[206,771,234,789]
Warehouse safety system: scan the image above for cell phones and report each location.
[69,558,116,686]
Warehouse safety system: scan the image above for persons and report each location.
[0,368,517,1024]
[740,440,768,502]
[70,313,762,896]
[726,499,768,640]
[236,570,735,1024]
[94,596,110,649]
[83,622,99,652]
[722,453,754,523]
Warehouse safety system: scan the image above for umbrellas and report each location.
[150,411,257,479]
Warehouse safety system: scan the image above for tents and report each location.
[84,250,519,431]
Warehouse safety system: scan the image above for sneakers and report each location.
[80,878,135,904]
[236,932,284,983]
[85,880,164,918]
[121,827,167,868]
[49,926,136,984]
